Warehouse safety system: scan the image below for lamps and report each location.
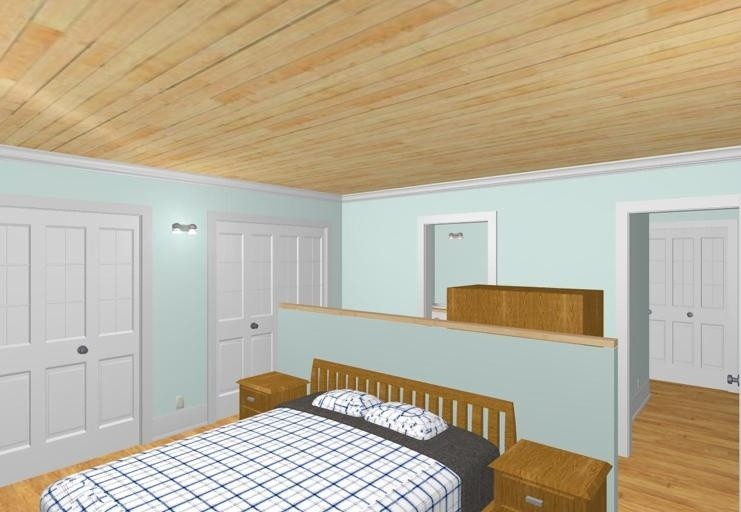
[171,222,199,234]
[448,233,464,240]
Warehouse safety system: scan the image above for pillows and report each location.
[363,401,449,441]
[311,388,383,417]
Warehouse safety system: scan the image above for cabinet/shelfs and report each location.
[447,284,603,334]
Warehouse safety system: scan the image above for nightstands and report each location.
[236,372,308,420]
[487,438,613,511]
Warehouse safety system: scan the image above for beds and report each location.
[38,359,516,512]
